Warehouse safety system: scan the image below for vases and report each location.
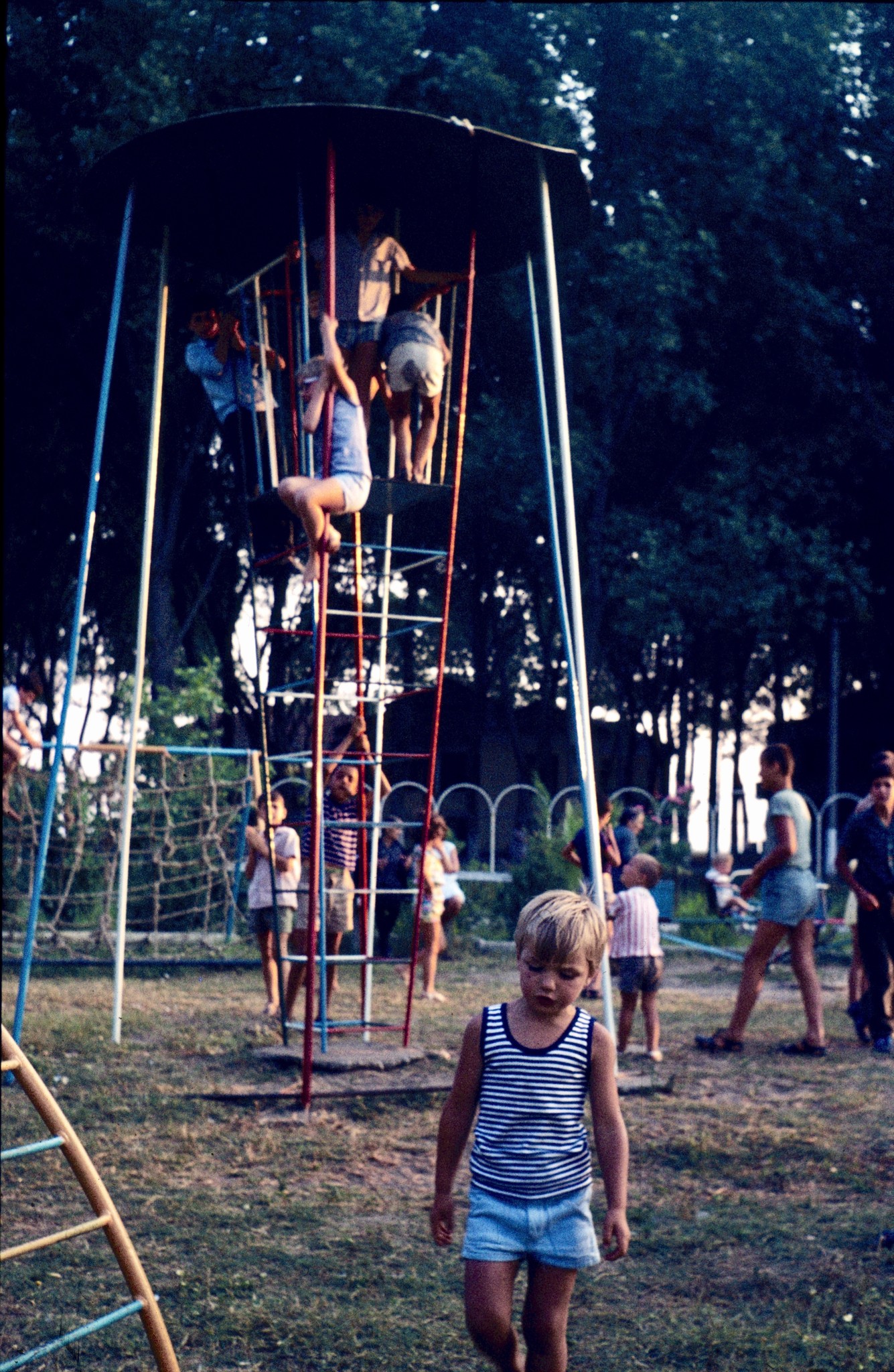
[645,880,673,920]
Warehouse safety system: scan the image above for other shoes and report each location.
[264,1002,346,1038]
[420,992,447,1004]
[694,1027,745,1053]
[780,1037,828,1056]
[872,1036,894,1055]
[846,1000,871,1042]
[395,963,411,985]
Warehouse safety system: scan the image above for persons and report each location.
[396,814,465,1004]
[366,814,412,958]
[561,744,894,1063]
[244,791,302,1015]
[2,673,43,821]
[430,889,630,1372]
[283,717,394,1033]
[186,203,450,585]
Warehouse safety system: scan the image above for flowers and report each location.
[634,781,701,877]
